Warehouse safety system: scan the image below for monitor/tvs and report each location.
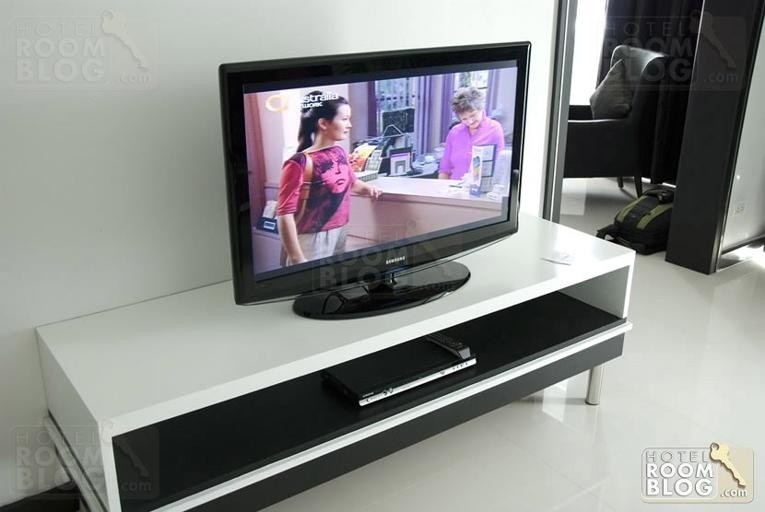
[219,41,532,320]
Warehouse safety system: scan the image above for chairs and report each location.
[563,45,670,198]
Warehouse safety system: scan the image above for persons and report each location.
[438,87,505,181]
[301,147,353,233]
[274,91,382,268]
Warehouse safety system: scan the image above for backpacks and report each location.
[596,185,676,255]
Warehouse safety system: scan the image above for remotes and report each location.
[425,333,471,360]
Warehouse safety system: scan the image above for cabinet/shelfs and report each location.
[35,211,637,512]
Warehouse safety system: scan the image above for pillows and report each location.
[589,59,632,120]
